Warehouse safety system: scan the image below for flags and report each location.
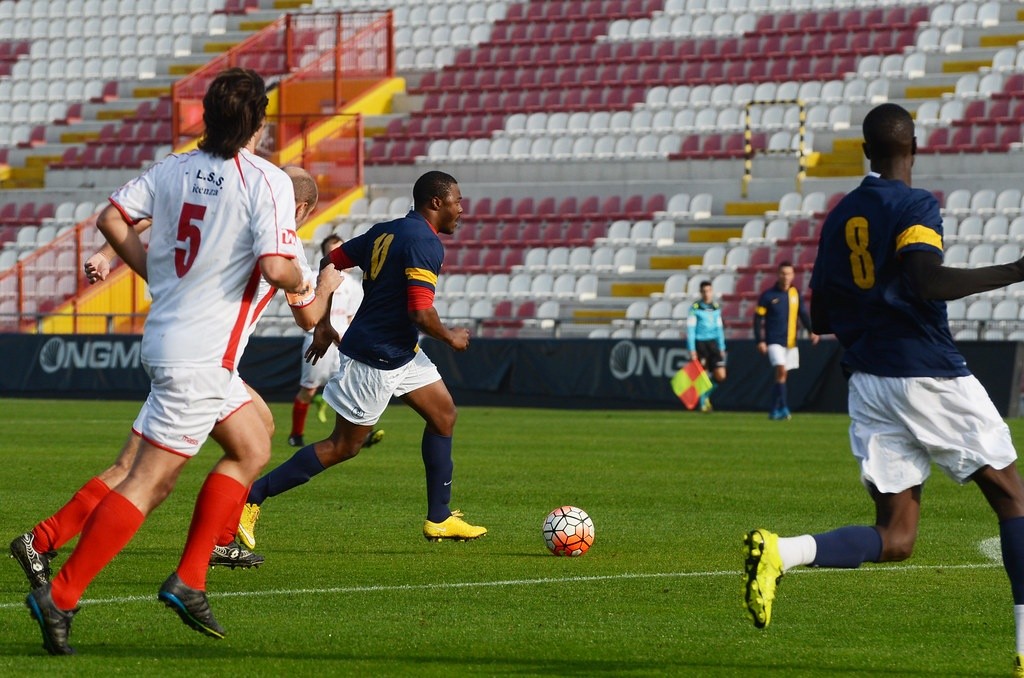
[669,359,713,411]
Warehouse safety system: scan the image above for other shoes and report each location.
[769,408,781,419]
[781,407,792,419]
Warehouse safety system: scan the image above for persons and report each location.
[753,260,820,421]
[233,170,488,549]
[10,68,386,656]
[741,104,1024,678]
[688,281,727,411]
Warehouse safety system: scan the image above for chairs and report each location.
[0,0,1023,345]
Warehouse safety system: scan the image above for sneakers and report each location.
[207,540,265,570]
[157,572,225,639]
[314,394,327,422]
[362,429,384,449]
[1012,653,1024,678]
[24,582,81,656]
[288,432,303,446]
[424,508,487,541]
[237,502,260,550]
[742,527,786,630]
[9,531,58,590]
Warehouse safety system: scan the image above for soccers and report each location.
[541,505,597,557]
